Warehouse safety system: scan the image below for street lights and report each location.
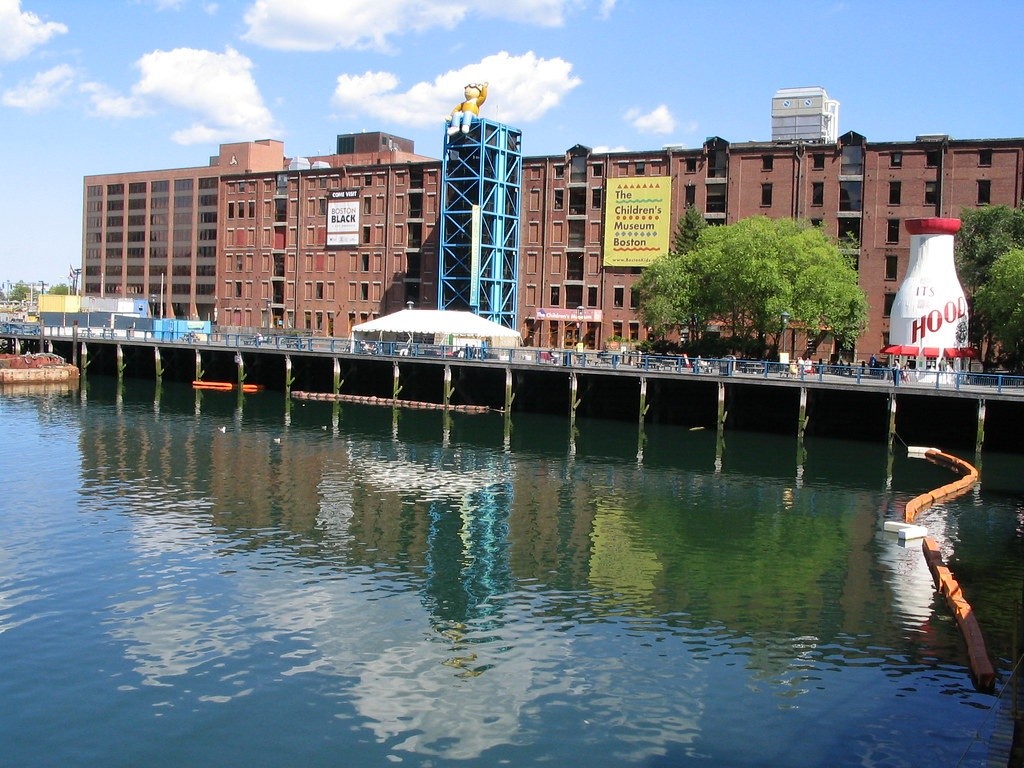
[406,300,415,356]
[576,305,586,364]
[149,294,157,331]
[266,299,273,344]
[779,310,792,377]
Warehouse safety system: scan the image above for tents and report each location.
[350,309,520,361]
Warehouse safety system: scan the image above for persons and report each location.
[400,344,411,355]
[293,336,302,349]
[868,353,876,374]
[902,362,910,383]
[833,359,851,375]
[255,332,263,345]
[549,348,560,365]
[603,341,608,351]
[790,357,812,378]
[697,355,728,376]
[445,81,489,135]
[458,344,477,359]
[893,360,900,387]
[363,343,379,354]
[939,358,947,372]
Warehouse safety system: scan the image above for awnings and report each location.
[944,348,976,357]
[879,345,901,355]
[924,348,939,357]
[900,346,919,356]
